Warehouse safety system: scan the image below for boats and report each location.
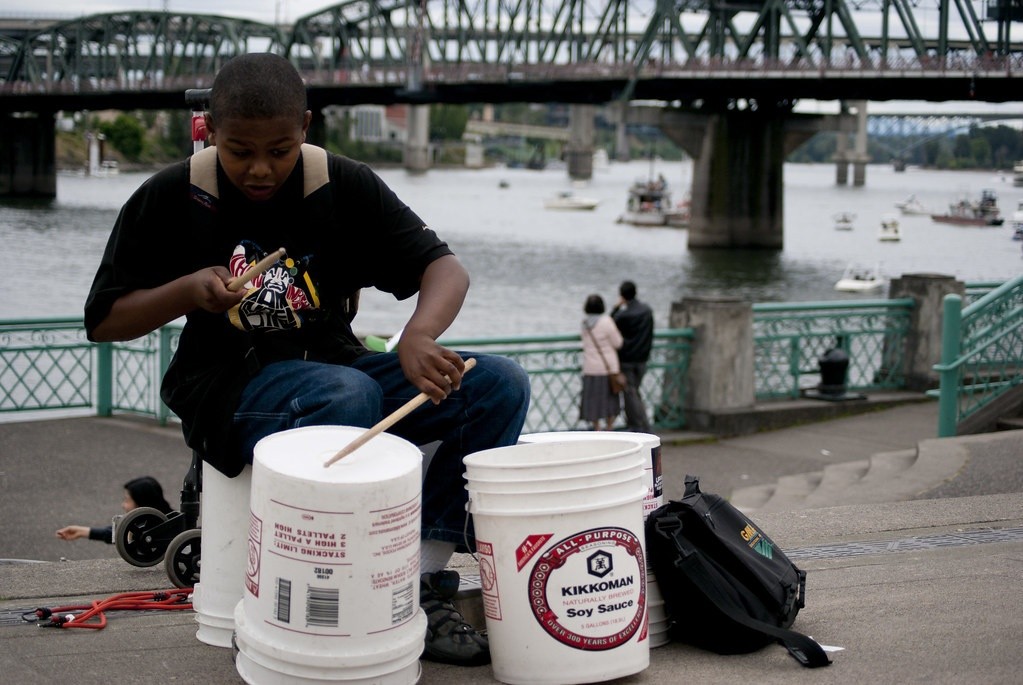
[879,220,902,243]
[832,273,883,291]
[662,202,691,225]
[928,190,1005,226]
[544,190,600,211]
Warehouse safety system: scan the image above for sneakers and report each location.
[420,570,491,667]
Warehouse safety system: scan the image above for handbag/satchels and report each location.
[644,474,833,668]
[610,372,625,395]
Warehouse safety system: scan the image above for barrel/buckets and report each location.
[517,431,663,648]
[193,460,252,648]
[234,424,428,685]
[462,438,649,685]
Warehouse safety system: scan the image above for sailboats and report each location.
[613,130,670,228]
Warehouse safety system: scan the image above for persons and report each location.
[56,476,175,545]
[84,53,531,667]
[579,281,654,433]
